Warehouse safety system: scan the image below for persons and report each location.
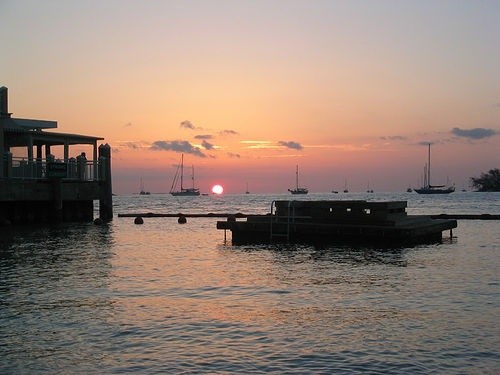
[76,152,87,178]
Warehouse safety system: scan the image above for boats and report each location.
[407,188,412,192]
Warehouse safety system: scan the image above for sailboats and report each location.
[413,143,455,194]
[288,165,308,194]
[366,181,374,193]
[343,178,349,193]
[170,154,200,196]
